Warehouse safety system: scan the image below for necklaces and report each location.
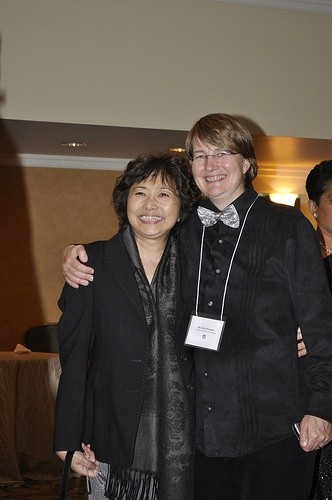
[318,240,332,254]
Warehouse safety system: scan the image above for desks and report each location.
[0,350,80,483]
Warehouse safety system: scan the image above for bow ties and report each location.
[197,203,240,228]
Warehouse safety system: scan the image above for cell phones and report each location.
[291,423,302,440]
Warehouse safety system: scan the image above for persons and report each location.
[51,151,202,500]
[59,111,332,500]
[293,159,332,500]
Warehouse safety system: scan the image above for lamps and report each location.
[268,193,299,208]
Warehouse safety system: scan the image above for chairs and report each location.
[24,323,61,354]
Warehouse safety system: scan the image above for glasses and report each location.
[188,150,241,165]
